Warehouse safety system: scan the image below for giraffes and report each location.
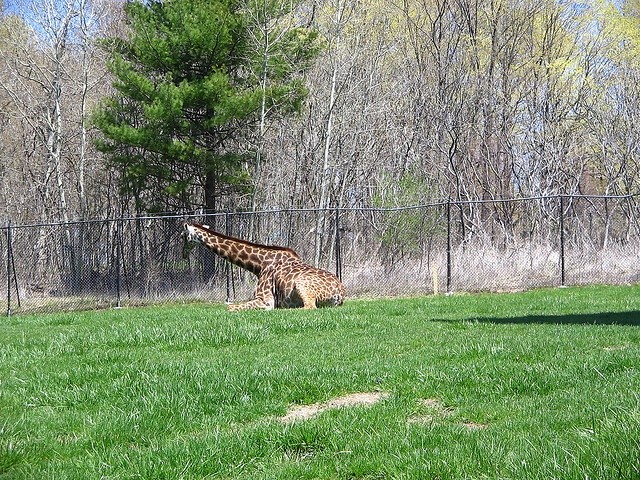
[179,217,347,312]
